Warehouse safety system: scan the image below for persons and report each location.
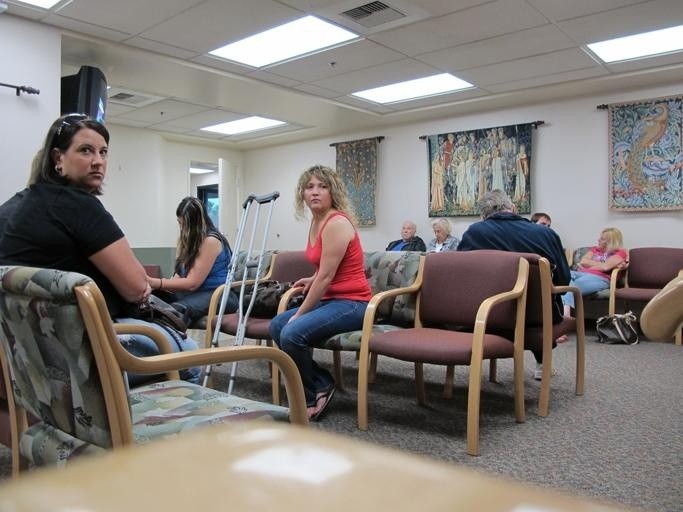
[427,219,460,252]
[531,213,551,228]
[386,221,426,251]
[147,198,240,330]
[0,113,202,389]
[269,166,373,421]
[456,190,571,380]
[556,228,627,342]
[430,127,529,212]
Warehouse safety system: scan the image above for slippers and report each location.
[314,384,337,420]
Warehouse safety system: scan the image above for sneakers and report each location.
[535,364,555,380]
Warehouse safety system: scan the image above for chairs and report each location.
[191,250,682,458]
[0,266,310,482]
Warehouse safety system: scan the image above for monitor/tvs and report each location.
[61,66,107,128]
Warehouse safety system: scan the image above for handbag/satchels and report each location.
[596,311,640,346]
[136,294,191,338]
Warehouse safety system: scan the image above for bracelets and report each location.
[158,278,162,290]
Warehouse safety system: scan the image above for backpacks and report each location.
[242,279,306,319]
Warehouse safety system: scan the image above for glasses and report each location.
[57,111,90,142]
